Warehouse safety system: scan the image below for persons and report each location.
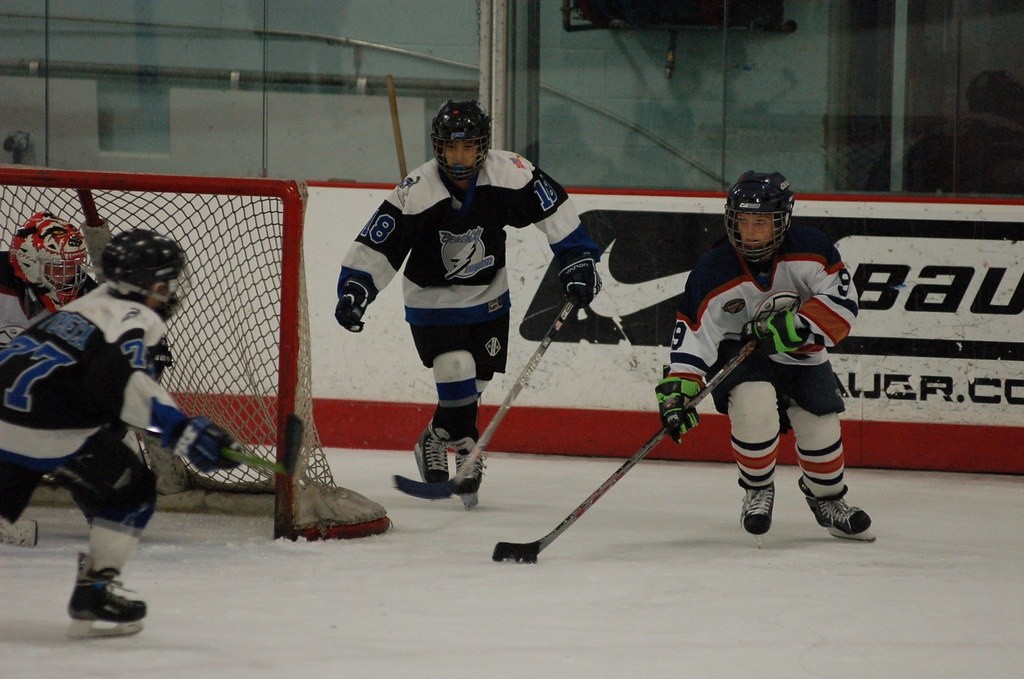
[655,172,876,540]
[334,100,602,511]
[0,212,241,638]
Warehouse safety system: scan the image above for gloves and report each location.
[740,310,802,357]
[654,377,700,443]
[169,414,244,477]
[558,256,602,309]
[335,277,371,332]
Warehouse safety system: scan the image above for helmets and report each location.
[430,97,492,181]
[726,171,795,237]
[8,211,88,314]
[102,230,186,319]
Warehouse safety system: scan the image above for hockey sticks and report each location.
[490,341,760,566]
[221,413,305,480]
[390,295,573,501]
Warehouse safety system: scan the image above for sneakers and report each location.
[414,418,453,486]
[66,550,147,637]
[797,475,876,542]
[736,477,775,550]
[445,427,486,509]
